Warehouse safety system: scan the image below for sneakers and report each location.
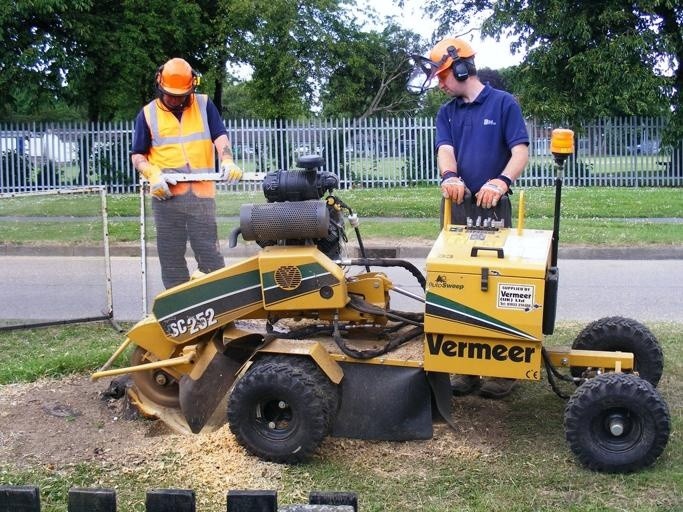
[450,375,517,398]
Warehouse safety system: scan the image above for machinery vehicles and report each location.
[87,127,673,476]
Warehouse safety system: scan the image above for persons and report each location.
[130,58,244,291]
[430,38,532,399]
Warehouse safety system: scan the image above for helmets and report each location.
[427,36,475,79]
[156,58,202,98]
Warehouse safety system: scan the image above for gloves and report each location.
[439,169,474,206]
[141,165,178,202]
[475,174,512,210]
[219,158,243,185]
